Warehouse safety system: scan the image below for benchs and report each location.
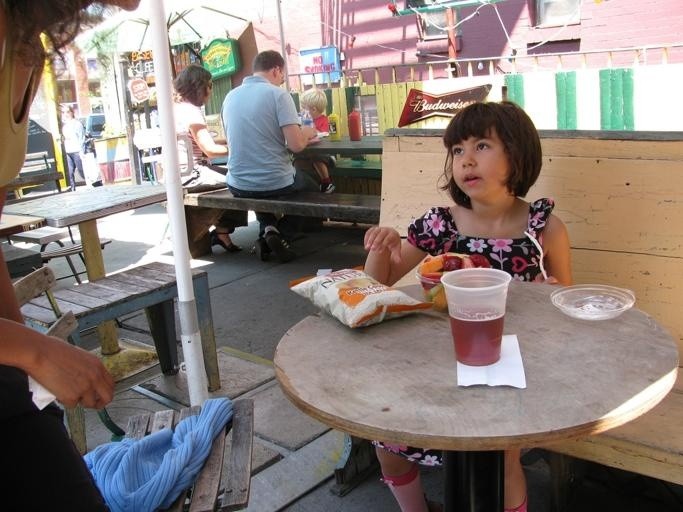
[161,184,382,261]
[16,261,222,457]
[292,160,382,179]
[330,121,683,498]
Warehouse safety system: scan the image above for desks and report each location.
[272,280,679,512]
[204,133,384,164]
[0,182,188,383]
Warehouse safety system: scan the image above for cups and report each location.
[441,268,512,366]
[412,265,445,313]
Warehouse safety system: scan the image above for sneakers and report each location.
[319,182,337,194]
[255,232,295,262]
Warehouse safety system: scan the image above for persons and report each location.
[364,99,572,511]
[169,64,248,252]
[60,105,103,190]
[219,50,319,264]
[0,0,138,511]
[299,88,338,195]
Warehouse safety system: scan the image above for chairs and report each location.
[0,150,112,283]
[11,266,254,512]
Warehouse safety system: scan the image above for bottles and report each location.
[348,112,362,142]
[326,113,341,141]
[302,110,315,129]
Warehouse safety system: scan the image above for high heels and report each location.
[209,230,243,253]
